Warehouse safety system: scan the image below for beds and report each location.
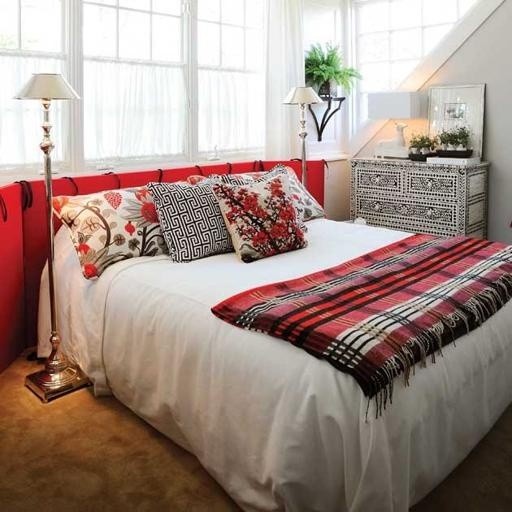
[38,162,512,511]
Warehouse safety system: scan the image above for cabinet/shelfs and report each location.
[349,156,490,239]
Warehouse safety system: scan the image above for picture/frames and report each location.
[427,83,486,161]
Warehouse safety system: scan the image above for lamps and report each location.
[281,86,324,189]
[11,73,93,403]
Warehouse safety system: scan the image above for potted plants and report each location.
[409,136,420,155]
[304,43,362,97]
[452,125,468,152]
[440,128,449,150]
[420,133,432,154]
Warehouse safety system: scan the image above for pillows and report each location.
[210,177,308,262]
[146,175,234,263]
[48,185,167,280]
[256,161,327,223]
